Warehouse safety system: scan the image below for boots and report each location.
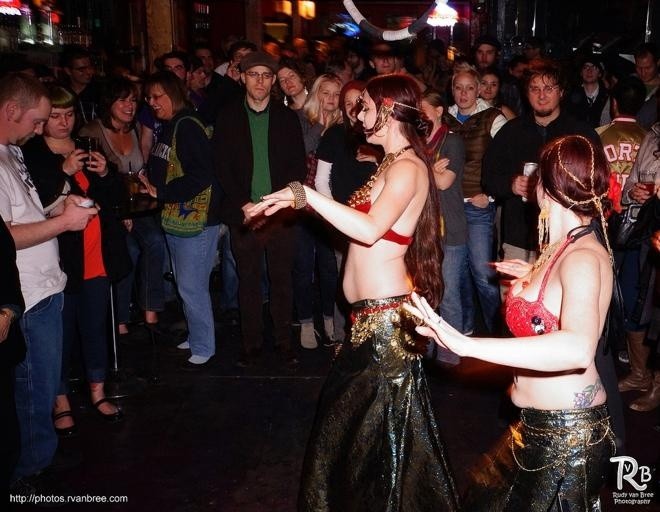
[617,332,654,393]
[629,372,660,413]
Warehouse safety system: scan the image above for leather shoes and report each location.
[91,398,123,423]
[54,407,77,437]
[168,340,215,373]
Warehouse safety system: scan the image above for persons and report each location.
[0,27,660,512]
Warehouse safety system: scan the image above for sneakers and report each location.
[300,323,317,349]
[324,318,337,342]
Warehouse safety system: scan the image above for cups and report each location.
[640,170,657,195]
[521,161,539,202]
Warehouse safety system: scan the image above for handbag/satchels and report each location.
[162,148,212,238]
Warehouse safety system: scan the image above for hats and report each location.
[237,50,279,75]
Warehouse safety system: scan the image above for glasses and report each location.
[142,93,165,102]
[248,70,271,78]
